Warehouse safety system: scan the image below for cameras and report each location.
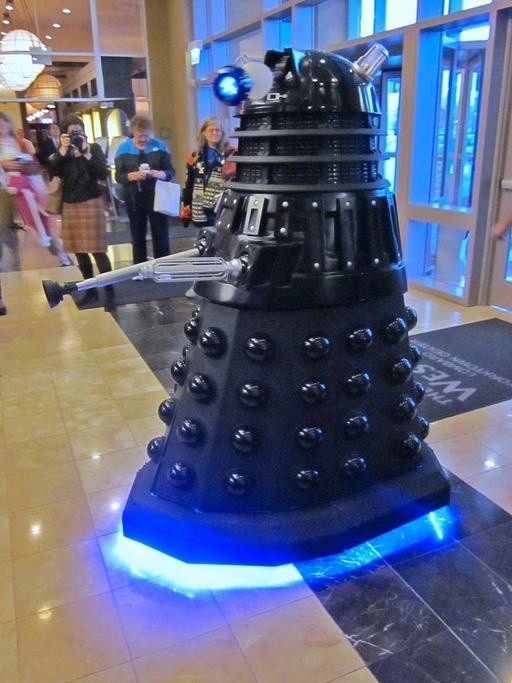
[68,129,87,153]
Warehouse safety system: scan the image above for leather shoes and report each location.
[104,288,115,312]
[77,288,97,307]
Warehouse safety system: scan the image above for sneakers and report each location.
[184,287,198,298]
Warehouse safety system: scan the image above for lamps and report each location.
[0,26,64,123]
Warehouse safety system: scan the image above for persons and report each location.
[114,113,176,264]
[1,111,73,271]
[17,127,36,154]
[49,113,117,311]
[177,115,237,297]
[39,121,64,172]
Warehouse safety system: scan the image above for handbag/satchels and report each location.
[44,177,64,215]
[154,180,181,216]
